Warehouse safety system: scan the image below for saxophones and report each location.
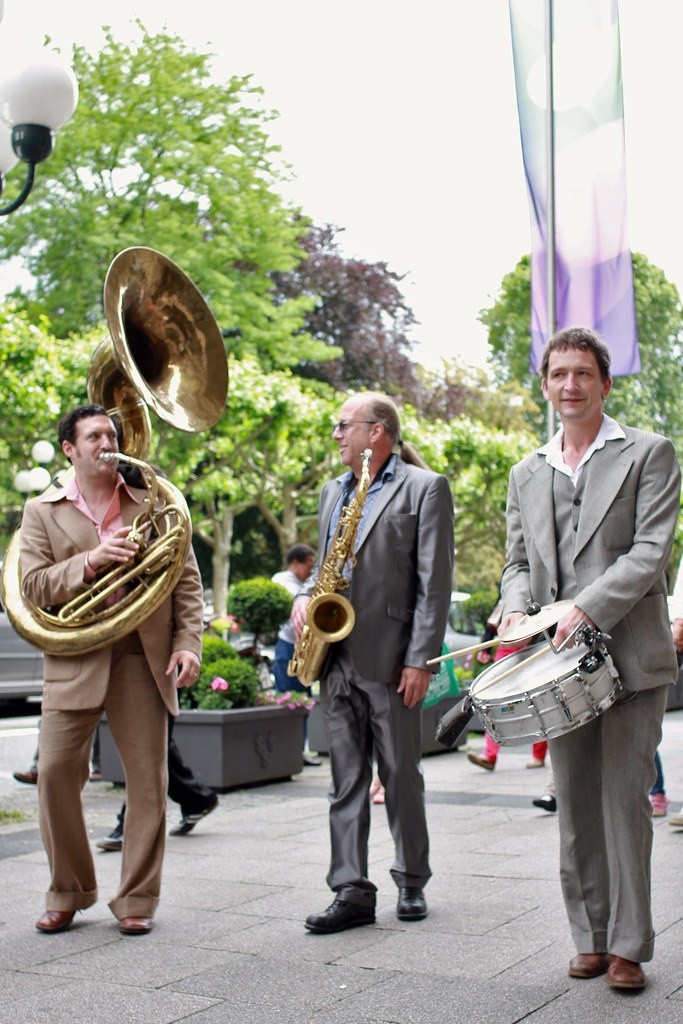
[287,449,373,688]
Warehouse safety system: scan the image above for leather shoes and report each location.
[397,887,427,918]
[568,953,609,977]
[608,953,646,988]
[304,900,375,931]
[36,911,75,931]
[120,916,152,933]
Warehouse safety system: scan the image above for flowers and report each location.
[465,650,491,676]
[254,684,316,711]
[199,678,234,712]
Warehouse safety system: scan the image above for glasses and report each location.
[333,420,376,431]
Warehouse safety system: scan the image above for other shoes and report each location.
[526,758,544,768]
[302,750,322,765]
[13,771,37,784]
[533,794,557,811]
[373,787,384,803]
[668,809,683,824]
[89,771,102,782]
[652,793,666,816]
[468,752,494,769]
[369,779,381,795]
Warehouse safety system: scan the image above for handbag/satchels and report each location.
[435,695,476,748]
[422,643,462,710]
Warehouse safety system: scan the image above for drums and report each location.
[469,640,628,747]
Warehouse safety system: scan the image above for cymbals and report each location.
[499,599,576,644]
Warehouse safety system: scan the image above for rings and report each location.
[193,672,198,677]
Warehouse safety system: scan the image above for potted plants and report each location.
[98,577,309,792]
[307,591,511,760]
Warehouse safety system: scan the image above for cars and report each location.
[203,589,275,688]
[0,606,45,696]
[443,591,484,670]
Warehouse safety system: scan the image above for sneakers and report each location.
[169,801,218,836]
[96,829,122,850]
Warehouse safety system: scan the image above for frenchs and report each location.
[0,246,228,656]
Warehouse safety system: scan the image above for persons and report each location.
[11,403,220,934]
[272,544,322,765]
[466,323,683,988]
[302,391,455,935]
[369,776,385,803]
